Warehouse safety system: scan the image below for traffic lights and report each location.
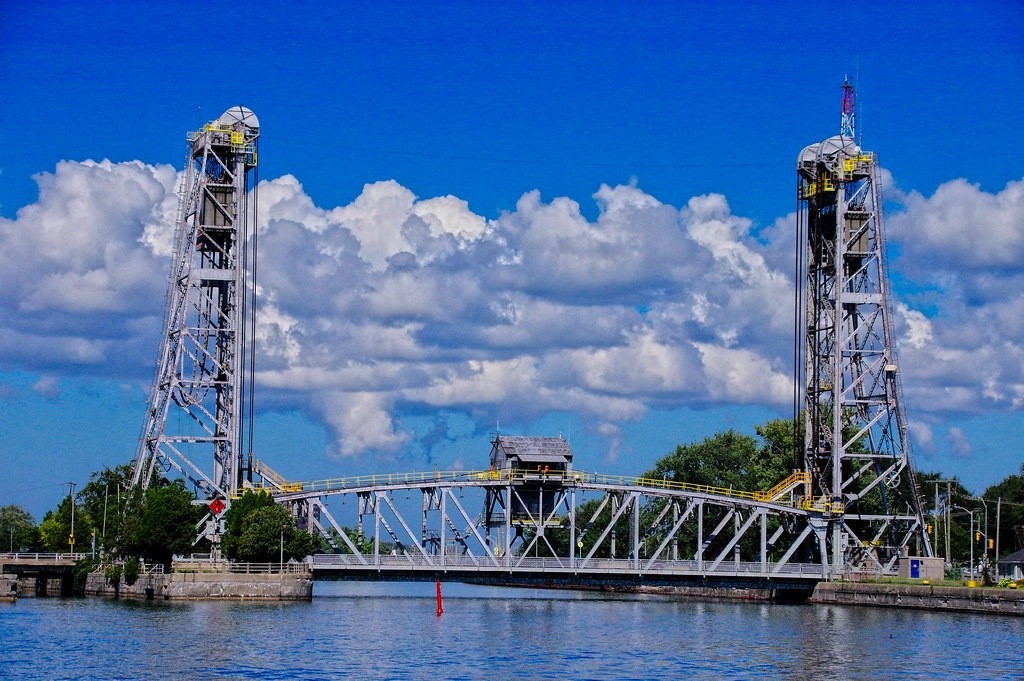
[976,533,980,541]
[989,538,994,550]
[928,526,933,534]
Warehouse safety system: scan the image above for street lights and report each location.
[953,506,974,581]
[977,497,988,554]
